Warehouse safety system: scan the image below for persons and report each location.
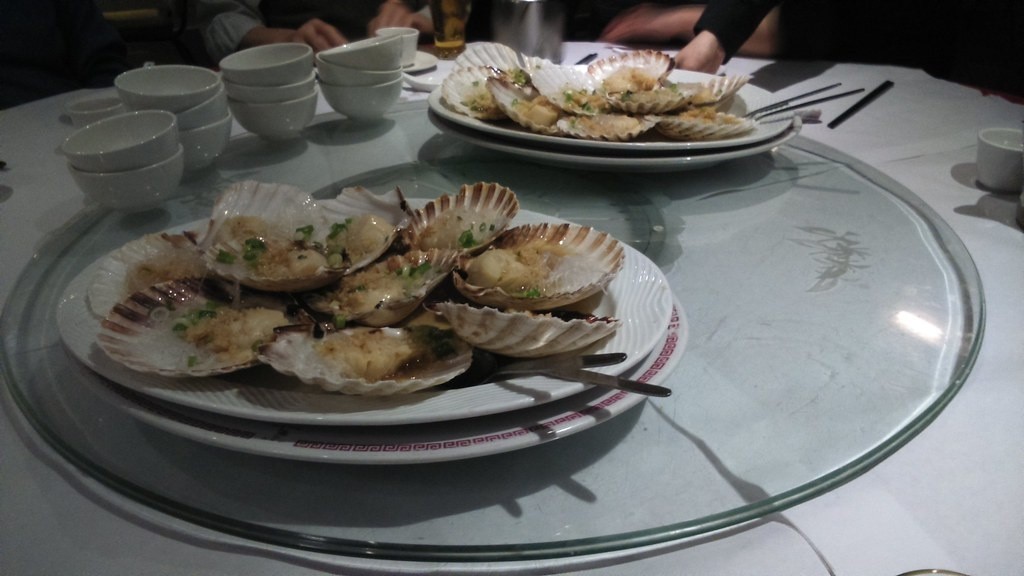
[597,0,1024,98]
[0,1,134,110]
[196,1,471,63]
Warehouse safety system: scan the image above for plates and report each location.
[427,68,797,151]
[57,297,689,464]
[401,50,436,74]
[56,198,676,429]
[426,106,804,172]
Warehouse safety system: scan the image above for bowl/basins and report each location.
[64,37,405,213]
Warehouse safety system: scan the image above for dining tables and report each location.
[0,42,1024,576]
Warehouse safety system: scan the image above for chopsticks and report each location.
[828,80,894,130]
[575,53,597,65]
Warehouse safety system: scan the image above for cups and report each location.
[374,27,420,67]
[974,127,1024,192]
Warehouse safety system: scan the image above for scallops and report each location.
[87,179,628,395]
[441,43,760,141]
